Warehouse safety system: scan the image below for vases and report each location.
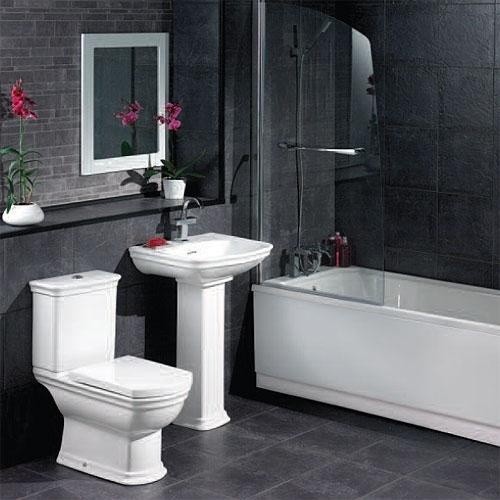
[0,78,46,214]
[142,103,208,183]
[112,99,144,156]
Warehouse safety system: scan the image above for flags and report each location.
[163,177,187,200]
[2,203,45,226]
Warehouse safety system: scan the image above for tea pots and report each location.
[81,32,168,175]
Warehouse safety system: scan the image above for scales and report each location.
[251,262,499,448]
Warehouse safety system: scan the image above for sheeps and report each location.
[181,197,202,219]
